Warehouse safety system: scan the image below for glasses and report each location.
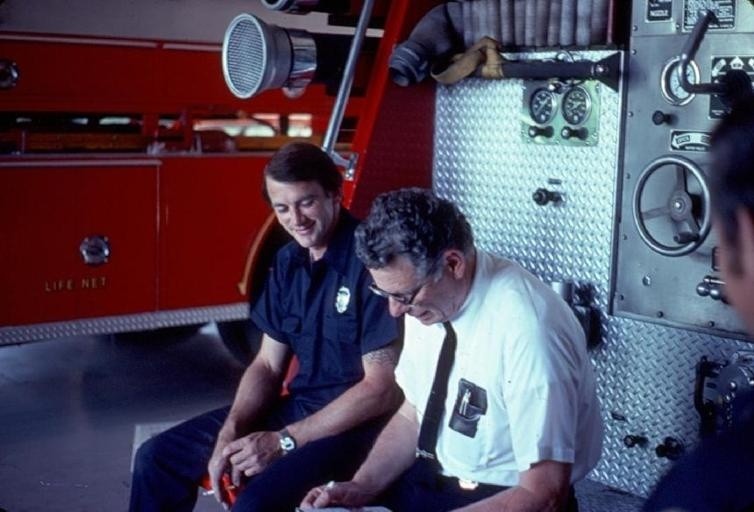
[366,253,441,305]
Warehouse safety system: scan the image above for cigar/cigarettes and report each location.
[202,485,236,496]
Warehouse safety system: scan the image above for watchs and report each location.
[278,428,297,456]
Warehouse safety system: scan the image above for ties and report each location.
[416,319,457,471]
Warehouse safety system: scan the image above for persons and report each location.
[642,101,754,511]
[129,143,403,511]
[294,187,604,512]
